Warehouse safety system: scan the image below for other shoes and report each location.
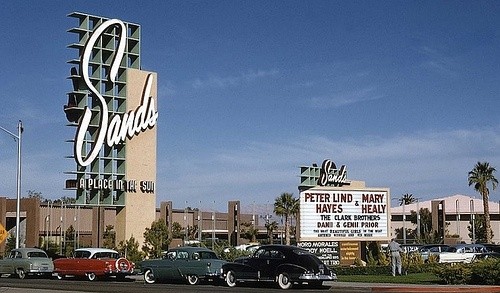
[397,274,402,276]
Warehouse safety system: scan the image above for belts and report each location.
[390,250,399,252]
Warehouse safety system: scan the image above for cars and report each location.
[139,246,232,286]
[383,244,493,264]
[53,247,136,281]
[0,248,54,280]
[223,243,338,290]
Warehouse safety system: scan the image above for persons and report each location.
[388,238,403,276]
